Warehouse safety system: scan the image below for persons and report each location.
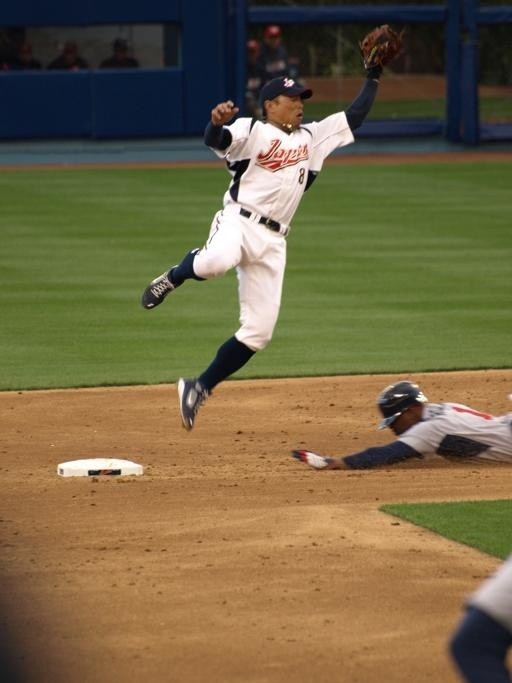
[99,36,139,68]
[1,40,43,68]
[46,40,88,69]
[244,24,303,91]
[141,22,406,430]
[291,379,512,470]
[245,38,260,54]
[449,553,512,682]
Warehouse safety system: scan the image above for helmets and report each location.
[377,380,423,430]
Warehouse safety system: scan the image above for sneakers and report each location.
[177,378,210,430]
[142,264,183,310]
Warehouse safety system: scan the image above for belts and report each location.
[238,209,287,236]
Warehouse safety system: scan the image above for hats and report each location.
[259,78,313,102]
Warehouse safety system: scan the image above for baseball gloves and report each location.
[359,25,403,68]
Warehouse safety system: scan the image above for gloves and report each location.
[291,449,333,470]
